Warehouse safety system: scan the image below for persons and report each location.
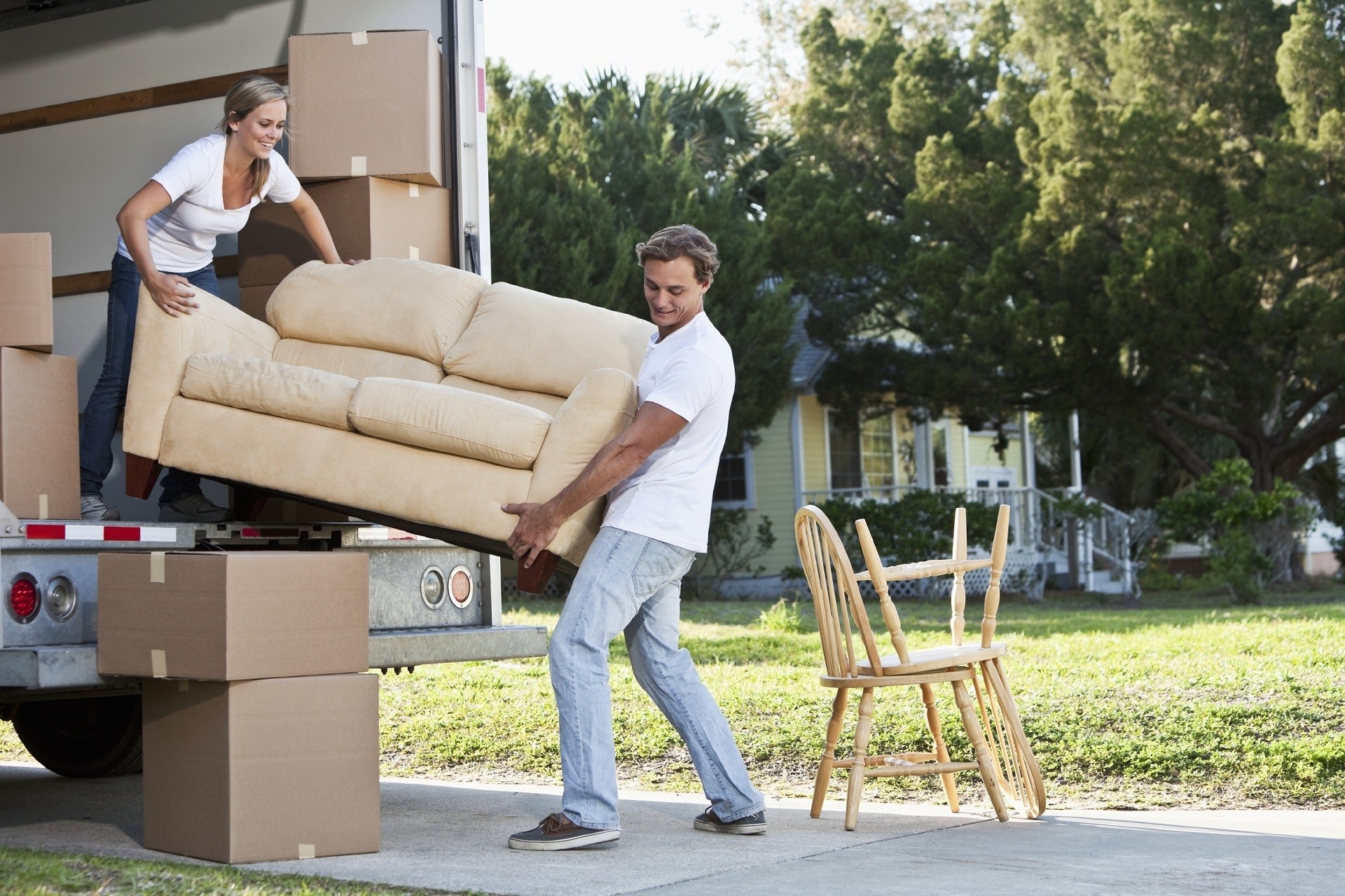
[78,76,365,525]
[501,224,768,851]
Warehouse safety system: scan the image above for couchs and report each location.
[121,258,660,592]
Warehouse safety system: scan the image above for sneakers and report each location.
[81,494,121,520]
[693,805,767,834]
[157,492,229,522]
[508,813,620,851]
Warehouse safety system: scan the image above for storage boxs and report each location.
[0,232,54,354]
[97,552,370,682]
[0,346,81,520]
[237,28,451,321]
[141,673,380,865]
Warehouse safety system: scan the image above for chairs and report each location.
[793,505,1047,831]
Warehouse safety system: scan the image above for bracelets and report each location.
[338,261,344,263]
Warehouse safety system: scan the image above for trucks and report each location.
[0,0,554,782]
